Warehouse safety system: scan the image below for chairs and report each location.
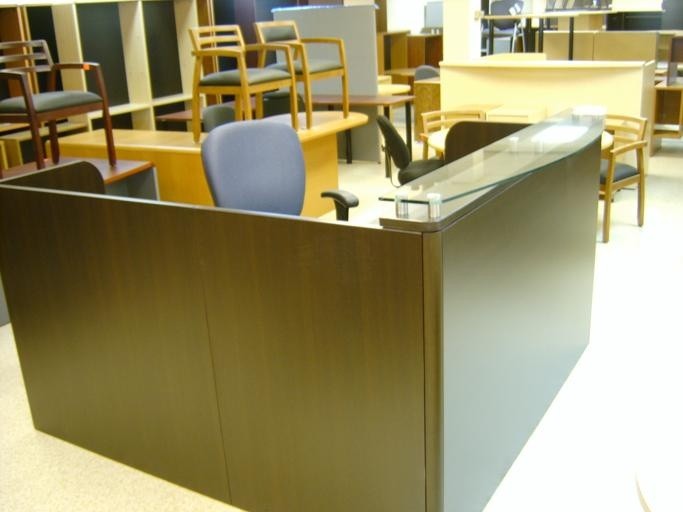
[483,0,527,54]
[186,19,349,143]
[599,112,647,243]
[199,120,360,228]
[0,39,118,170]
[374,108,486,190]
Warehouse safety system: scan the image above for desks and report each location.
[43,109,369,219]
[0,156,160,207]
[156,90,417,178]
